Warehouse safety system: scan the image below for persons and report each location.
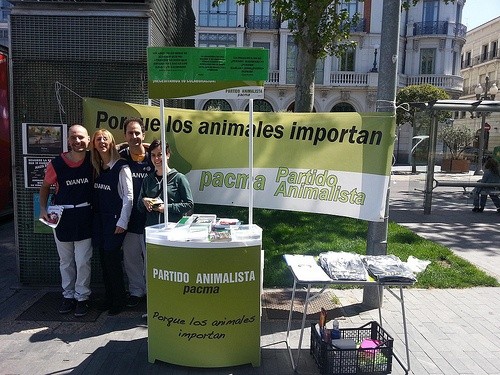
[137,139,194,318]
[39,125,96,317]
[119,118,155,306]
[90,129,133,316]
[471,156,500,212]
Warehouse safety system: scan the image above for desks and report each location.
[283,254,418,374]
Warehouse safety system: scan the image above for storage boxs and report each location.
[309,321,395,375]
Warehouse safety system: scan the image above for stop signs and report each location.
[484,122,491,131]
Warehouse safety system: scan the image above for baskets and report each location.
[311,321,394,375]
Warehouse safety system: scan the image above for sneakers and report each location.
[59,298,75,314]
[75,300,89,316]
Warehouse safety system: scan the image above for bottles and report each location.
[330,319,341,340]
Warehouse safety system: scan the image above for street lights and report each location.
[473,74,498,175]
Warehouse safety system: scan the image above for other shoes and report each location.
[496,209,500,213]
[127,296,140,307]
[472,207,484,213]
[108,305,123,316]
[98,303,111,312]
